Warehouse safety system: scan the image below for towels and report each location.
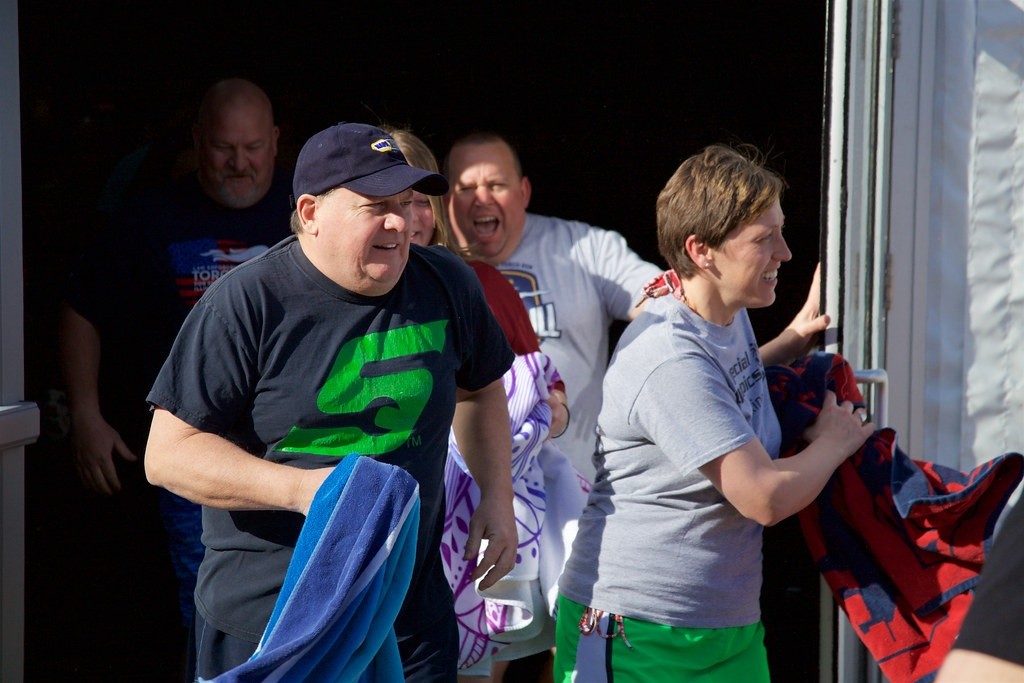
[194,452,424,683]
[761,350,1024,683]
[437,348,599,681]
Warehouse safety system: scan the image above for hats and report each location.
[291,122,449,204]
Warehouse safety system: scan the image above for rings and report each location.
[853,409,868,423]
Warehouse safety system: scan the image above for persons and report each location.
[143,121,519,681]
[437,126,670,682]
[549,142,877,683]
[57,77,295,628]
[379,127,570,680]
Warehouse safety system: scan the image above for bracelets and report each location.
[551,402,571,439]
